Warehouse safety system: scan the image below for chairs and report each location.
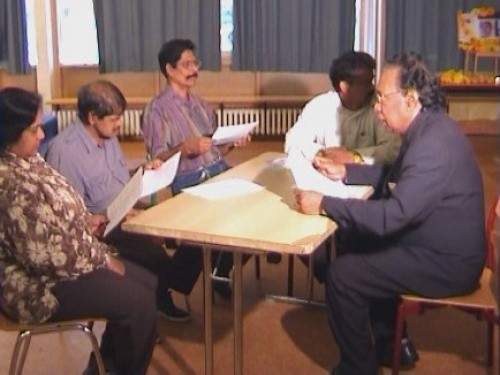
[392,195,500,375]
[0,106,260,375]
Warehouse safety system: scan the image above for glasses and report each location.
[376,90,402,103]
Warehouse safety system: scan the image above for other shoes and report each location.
[382,340,420,369]
[157,291,190,321]
[205,272,237,308]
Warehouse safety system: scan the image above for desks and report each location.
[120,150,381,375]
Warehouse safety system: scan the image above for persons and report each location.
[140,39,251,295]
[0,85,163,375]
[45,80,191,322]
[292,51,488,374]
[284,50,405,285]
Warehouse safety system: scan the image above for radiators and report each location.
[52,107,304,136]
